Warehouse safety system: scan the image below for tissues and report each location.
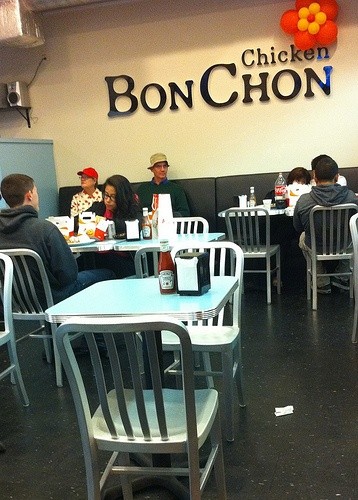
[124,219,141,240]
[174,252,212,296]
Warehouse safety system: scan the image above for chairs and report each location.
[301,203,358,343]
[0,192,288,500]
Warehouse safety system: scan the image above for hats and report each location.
[77,167,98,181]
[147,152,171,169]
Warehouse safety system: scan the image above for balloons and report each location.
[279,0,338,51]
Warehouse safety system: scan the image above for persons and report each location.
[0,174,123,357]
[72,154,190,280]
[262,154,358,294]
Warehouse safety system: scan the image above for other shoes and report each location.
[331,276,350,290]
[310,281,331,294]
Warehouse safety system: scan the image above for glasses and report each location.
[79,175,89,179]
[101,191,117,202]
[154,164,168,169]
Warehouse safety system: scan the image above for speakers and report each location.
[7,80,30,107]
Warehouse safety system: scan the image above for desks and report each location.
[217,205,286,246]
[69,237,122,271]
[112,231,227,283]
[44,275,239,499]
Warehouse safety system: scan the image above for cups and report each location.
[263,199,272,211]
[239,194,247,208]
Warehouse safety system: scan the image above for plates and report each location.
[67,239,95,246]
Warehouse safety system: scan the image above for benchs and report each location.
[57,167,358,279]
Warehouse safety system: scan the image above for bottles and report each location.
[148,193,158,221]
[274,173,287,209]
[158,238,176,295]
[249,186,257,207]
[141,208,153,239]
